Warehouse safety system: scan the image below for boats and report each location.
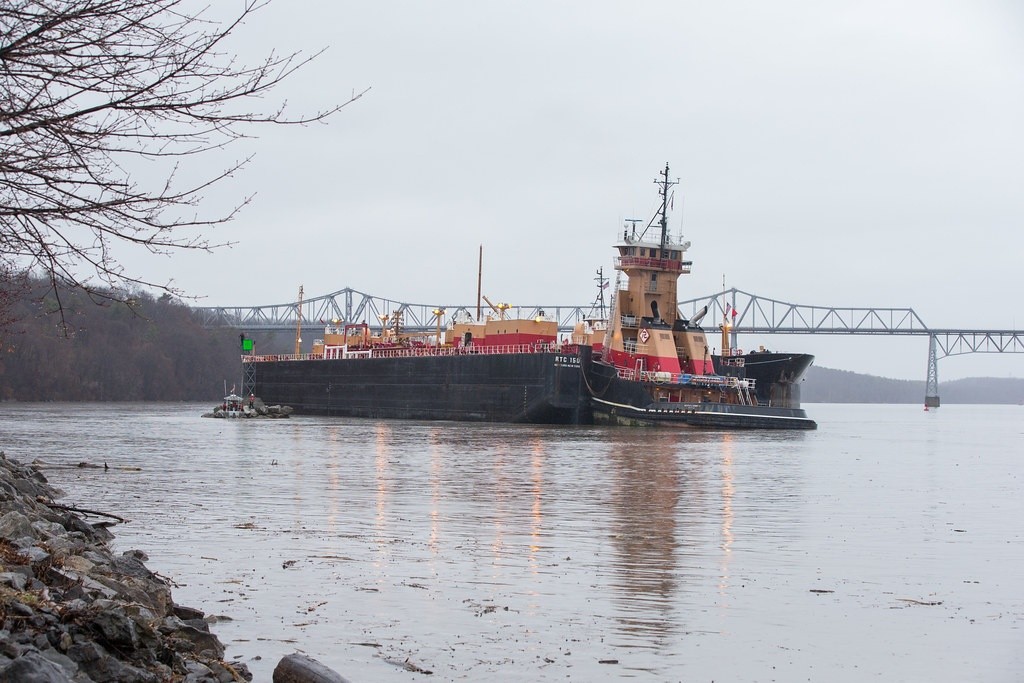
[235,160,818,430]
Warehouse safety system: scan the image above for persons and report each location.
[249,394,254,409]
[223,402,244,413]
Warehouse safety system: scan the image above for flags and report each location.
[731,308,738,319]
[724,302,731,318]
